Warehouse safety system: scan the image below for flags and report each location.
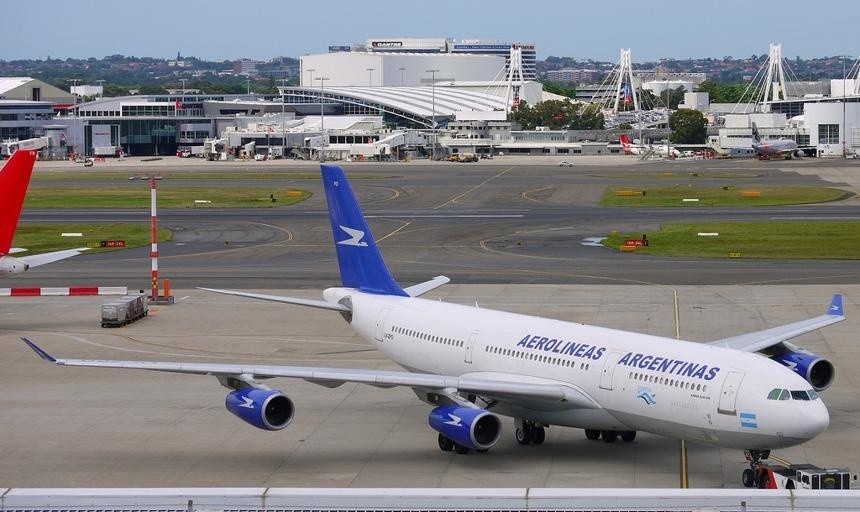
[174,98,185,111]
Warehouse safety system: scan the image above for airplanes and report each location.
[19,161,847,491]
[0,142,98,275]
[619,131,684,159]
[721,122,821,164]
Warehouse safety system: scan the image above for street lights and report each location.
[365,68,375,86]
[67,79,83,152]
[95,79,107,99]
[425,69,441,160]
[314,77,330,161]
[398,66,407,86]
[836,52,854,158]
[274,79,289,159]
[246,79,252,94]
[306,69,315,86]
[179,79,187,96]
[128,169,161,302]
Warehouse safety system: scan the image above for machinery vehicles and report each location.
[100,289,150,327]
[741,459,859,492]
